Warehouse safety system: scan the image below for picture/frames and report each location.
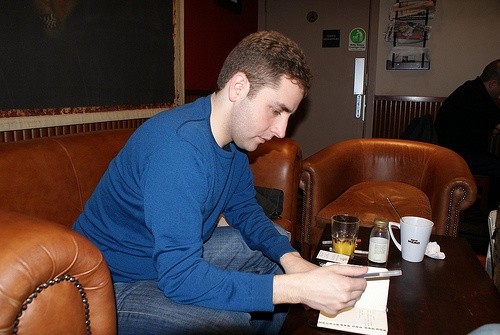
[0,0,185,131]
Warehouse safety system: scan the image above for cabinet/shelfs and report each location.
[386,0,431,70]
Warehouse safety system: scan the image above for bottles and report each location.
[367,217,390,264]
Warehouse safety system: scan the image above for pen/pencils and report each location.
[350,269,404,281]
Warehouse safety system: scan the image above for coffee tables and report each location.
[279,223,500,335]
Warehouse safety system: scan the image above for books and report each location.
[317,264,390,335]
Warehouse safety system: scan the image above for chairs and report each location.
[400,114,489,237]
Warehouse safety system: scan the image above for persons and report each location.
[72,30,368,335]
[431,60,500,212]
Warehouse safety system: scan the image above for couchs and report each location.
[0,126,302,335]
[299,137,477,264]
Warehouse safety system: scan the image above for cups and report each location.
[388,216,434,263]
[331,215,360,257]
